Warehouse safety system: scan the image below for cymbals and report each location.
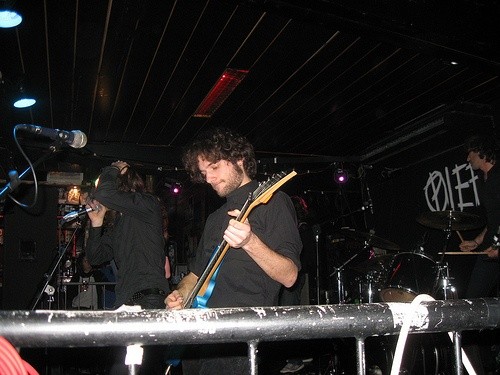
[415,209,484,230]
[341,228,400,251]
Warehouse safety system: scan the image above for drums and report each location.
[380,252,438,302]
[359,271,386,303]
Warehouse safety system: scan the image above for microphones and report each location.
[367,187,373,215]
[63,204,101,222]
[15,124,87,148]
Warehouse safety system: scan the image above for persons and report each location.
[459,137,500,259]
[163,126,303,375]
[74,251,171,310]
[278,197,313,373]
[86,161,171,375]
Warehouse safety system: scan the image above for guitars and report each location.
[163,168,297,367]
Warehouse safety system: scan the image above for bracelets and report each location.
[473,240,480,246]
